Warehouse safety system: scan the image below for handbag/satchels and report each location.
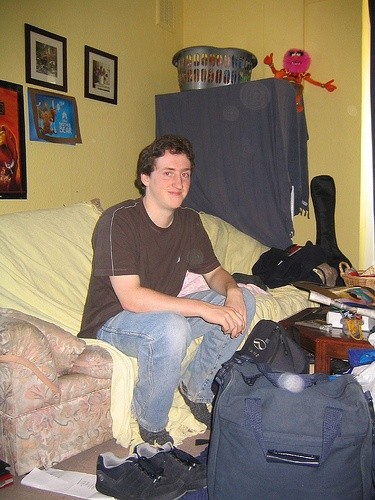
[206,361,375,500]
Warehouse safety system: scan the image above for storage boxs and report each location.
[326,310,375,332]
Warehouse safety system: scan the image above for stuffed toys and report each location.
[264,48,337,112]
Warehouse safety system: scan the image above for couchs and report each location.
[0,197,320,476]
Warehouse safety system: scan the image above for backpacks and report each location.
[244,320,309,373]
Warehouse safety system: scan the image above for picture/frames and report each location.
[24,22,68,92]
[83,44,118,106]
[0,79,28,200]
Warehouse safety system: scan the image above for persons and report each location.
[76,134,256,446]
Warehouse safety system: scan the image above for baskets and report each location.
[172,45,258,91]
[339,262,375,290]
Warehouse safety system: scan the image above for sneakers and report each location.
[96,452,187,500]
[133,442,208,492]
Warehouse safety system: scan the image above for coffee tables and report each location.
[278,305,375,374]
